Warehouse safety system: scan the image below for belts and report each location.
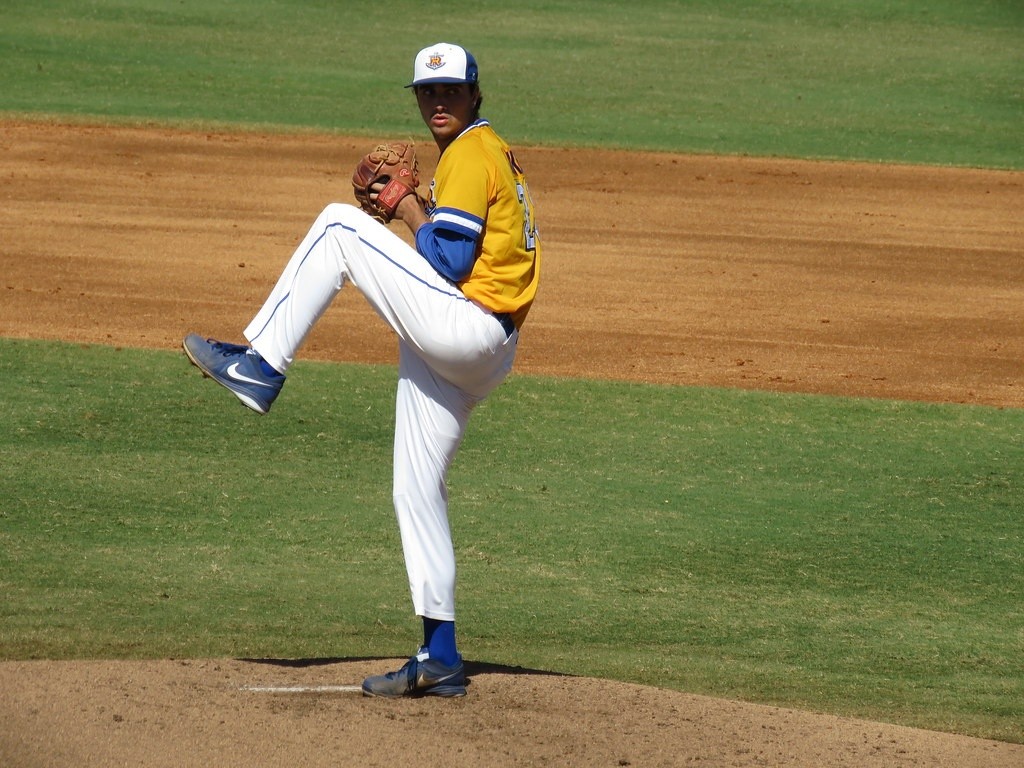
[492,311,519,344]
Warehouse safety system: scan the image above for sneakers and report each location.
[362,645,467,697]
[181,333,285,415]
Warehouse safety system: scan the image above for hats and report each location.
[404,43,478,88]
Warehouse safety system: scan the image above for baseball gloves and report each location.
[352,136,419,225]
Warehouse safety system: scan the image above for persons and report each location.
[181,42,542,699]
